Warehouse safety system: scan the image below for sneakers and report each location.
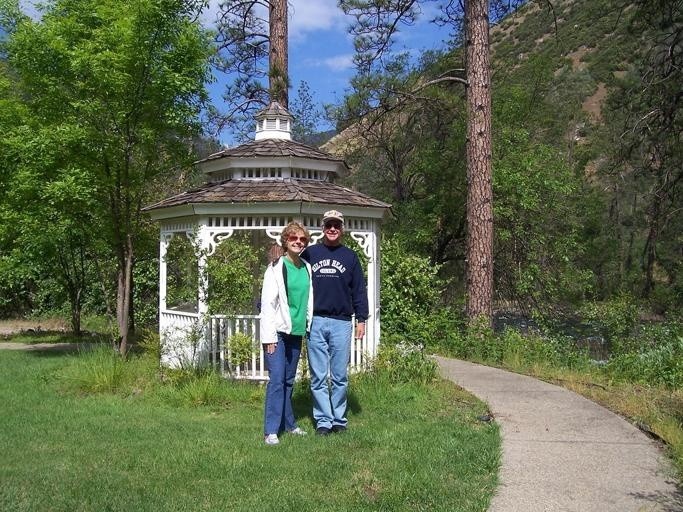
[332,425,345,433]
[264,434,279,445]
[317,427,331,436]
[287,428,307,436]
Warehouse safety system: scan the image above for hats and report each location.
[322,210,343,223]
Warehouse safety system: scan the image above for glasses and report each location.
[325,223,340,229]
[288,236,306,241]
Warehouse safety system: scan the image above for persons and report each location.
[258,223,315,445]
[270,210,369,435]
